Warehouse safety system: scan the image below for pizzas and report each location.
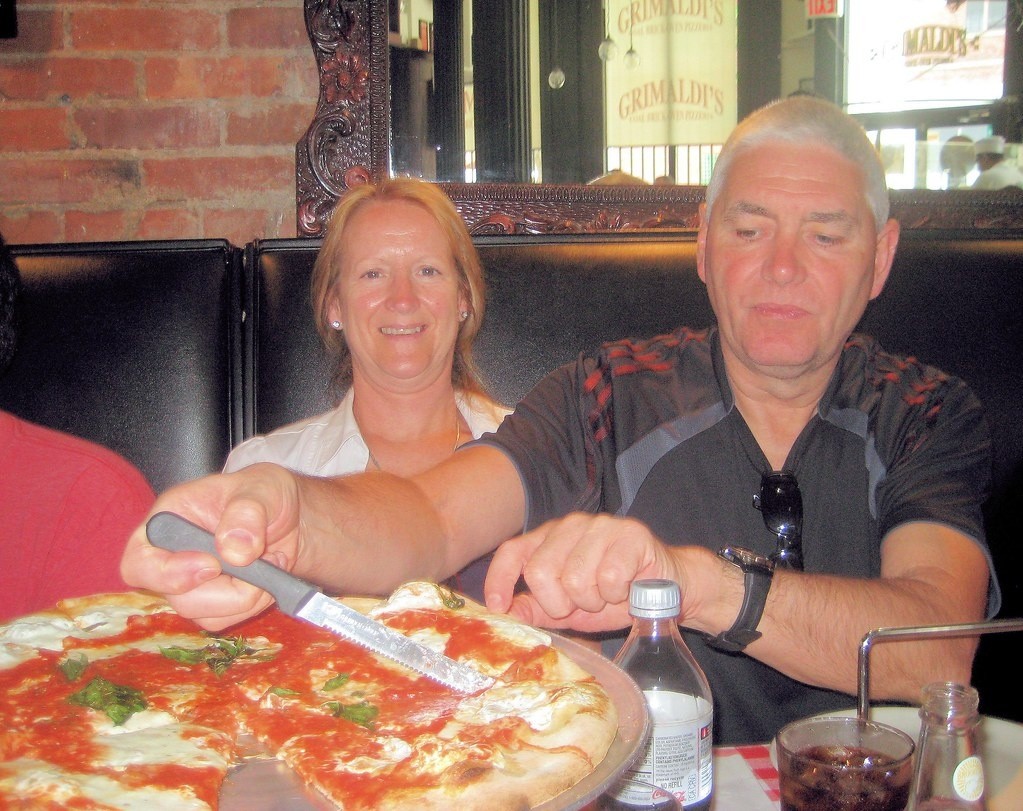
[0,579,618,811]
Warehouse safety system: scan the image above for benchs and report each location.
[0,236,1023,724]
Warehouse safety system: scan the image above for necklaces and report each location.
[454,420,459,450]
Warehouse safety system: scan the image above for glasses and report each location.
[760,471,804,573]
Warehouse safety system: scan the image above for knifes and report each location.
[146,510,494,694]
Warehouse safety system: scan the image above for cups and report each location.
[776,717,915,811]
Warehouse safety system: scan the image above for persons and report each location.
[223,179,515,605]
[118,95,1001,743]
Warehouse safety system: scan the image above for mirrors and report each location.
[298,1,1021,242]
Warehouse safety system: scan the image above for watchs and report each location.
[701,545,775,652]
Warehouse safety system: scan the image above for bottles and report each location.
[913,681,986,811]
[596,579,714,811]
